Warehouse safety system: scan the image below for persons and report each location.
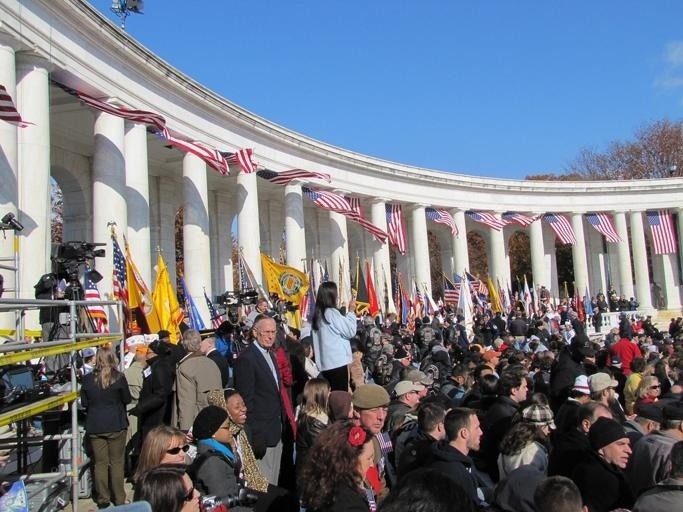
[437,364,467,404]
[631,375,660,402]
[385,380,424,431]
[533,475,588,512]
[79,342,132,509]
[183,406,254,511]
[156,330,185,362]
[582,416,635,511]
[376,461,479,512]
[419,406,492,512]
[127,338,176,456]
[352,382,390,505]
[623,355,645,414]
[393,401,446,479]
[133,423,188,483]
[124,345,148,455]
[206,387,268,492]
[175,328,222,443]
[232,314,300,512]
[245,297,267,326]
[79,347,96,378]
[406,370,434,398]
[548,401,614,484]
[621,401,683,501]
[35,272,70,383]
[555,374,593,441]
[471,373,499,409]
[586,372,618,406]
[352,284,683,385]
[296,377,330,449]
[294,418,374,512]
[215,320,233,358]
[131,461,200,512]
[311,281,357,393]
[300,339,318,378]
[458,363,492,405]
[480,371,528,480]
[495,402,555,512]
[631,440,682,511]
[198,329,229,388]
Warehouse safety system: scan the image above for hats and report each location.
[149,339,170,356]
[126,333,145,347]
[423,316,430,324]
[572,375,591,395]
[664,338,673,345]
[381,344,393,354]
[471,345,480,352]
[415,317,422,327]
[523,404,557,431]
[144,333,159,343]
[664,402,683,421]
[587,372,619,393]
[482,350,502,362]
[219,322,233,334]
[637,405,665,424]
[430,345,442,352]
[82,349,96,360]
[407,370,436,386]
[393,380,426,398]
[571,335,594,358]
[589,417,630,451]
[401,337,411,345]
[158,330,171,340]
[610,356,621,365]
[393,348,411,359]
[201,332,217,341]
[192,405,228,440]
[352,383,391,407]
[500,344,510,350]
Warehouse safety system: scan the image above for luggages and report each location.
[25,479,70,511]
[59,427,93,499]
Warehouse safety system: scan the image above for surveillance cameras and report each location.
[105,0,146,30]
[200,494,222,511]
[0,212,24,231]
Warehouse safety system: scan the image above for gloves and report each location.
[252,431,266,459]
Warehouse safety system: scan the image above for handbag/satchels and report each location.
[1,365,35,391]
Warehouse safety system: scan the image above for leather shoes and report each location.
[97,503,110,509]
[115,499,133,506]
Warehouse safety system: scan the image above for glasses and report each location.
[404,357,414,361]
[404,343,412,346]
[183,484,196,503]
[649,384,663,390]
[253,325,278,336]
[410,389,422,394]
[219,422,232,432]
[461,375,466,381]
[146,350,153,353]
[167,445,190,455]
[606,386,618,391]
[523,359,530,362]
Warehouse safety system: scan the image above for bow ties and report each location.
[267,347,272,353]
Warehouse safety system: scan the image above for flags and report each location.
[260,251,310,306]
[279,254,310,328]
[0,86,37,128]
[424,206,459,238]
[584,212,623,243]
[306,268,317,321]
[344,197,389,247]
[351,260,369,314]
[203,292,242,357]
[219,148,258,172]
[502,212,545,229]
[300,265,309,321]
[124,239,161,334]
[175,271,206,333]
[385,202,407,256]
[83,258,110,339]
[145,126,230,177]
[112,235,127,308]
[302,186,363,224]
[644,209,677,255]
[366,262,379,316]
[584,286,592,315]
[464,210,514,232]
[543,213,577,246]
[575,286,583,320]
[373,263,540,325]
[152,255,184,345]
[256,169,331,187]
[47,78,171,141]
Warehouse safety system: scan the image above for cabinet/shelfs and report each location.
[0,297,125,507]
